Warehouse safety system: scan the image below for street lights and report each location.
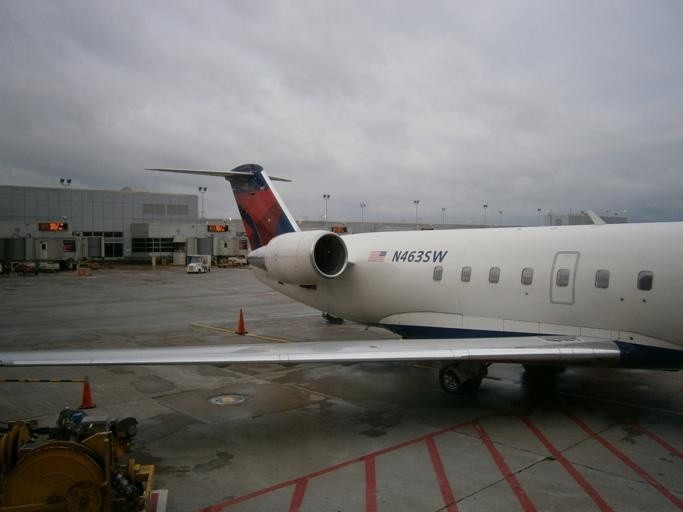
[323,193,541,223]
[198,186,206,219]
[57,176,71,189]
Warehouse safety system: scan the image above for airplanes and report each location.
[0,163,682,392]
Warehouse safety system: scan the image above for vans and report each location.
[185,251,212,275]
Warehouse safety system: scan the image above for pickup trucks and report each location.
[213,252,247,268]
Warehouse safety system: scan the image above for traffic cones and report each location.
[71,375,98,410]
[232,302,249,337]
